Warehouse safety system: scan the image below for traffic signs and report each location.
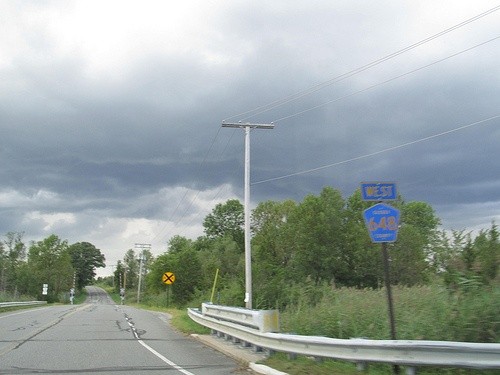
[361,181,398,201]
